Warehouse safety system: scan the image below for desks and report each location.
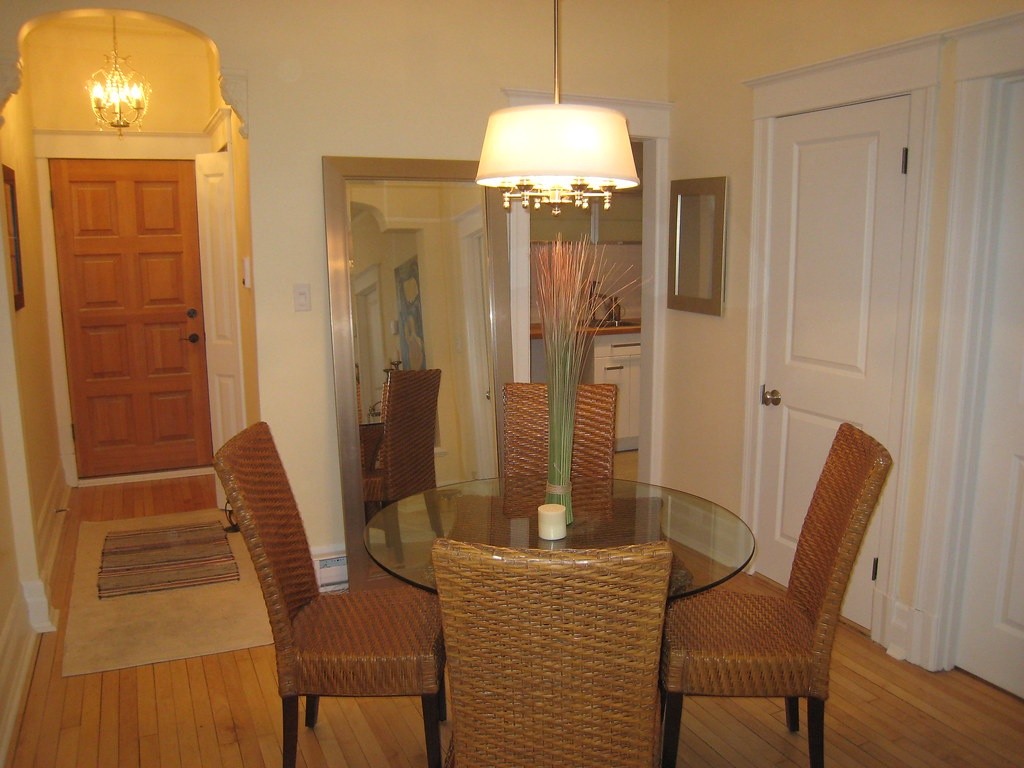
[363,475,756,599]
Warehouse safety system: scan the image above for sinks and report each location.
[584,319,642,328]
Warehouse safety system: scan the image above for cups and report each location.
[537,504,568,541]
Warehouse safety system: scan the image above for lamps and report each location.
[473,0,640,214]
[85,14,153,138]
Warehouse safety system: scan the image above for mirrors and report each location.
[323,157,515,593]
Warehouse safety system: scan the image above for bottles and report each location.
[608,296,621,320]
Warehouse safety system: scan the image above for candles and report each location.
[391,348,400,360]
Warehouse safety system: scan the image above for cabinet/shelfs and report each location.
[581,333,640,454]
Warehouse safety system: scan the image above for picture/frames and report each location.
[667,176,728,318]
[2,163,26,311]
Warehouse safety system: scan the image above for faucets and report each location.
[589,280,608,319]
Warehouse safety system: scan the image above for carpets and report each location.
[96,519,240,600]
[62,506,275,676]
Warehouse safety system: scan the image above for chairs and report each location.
[433,536,672,768]
[213,422,448,768]
[660,423,893,768]
[362,367,445,566]
[503,382,617,481]
